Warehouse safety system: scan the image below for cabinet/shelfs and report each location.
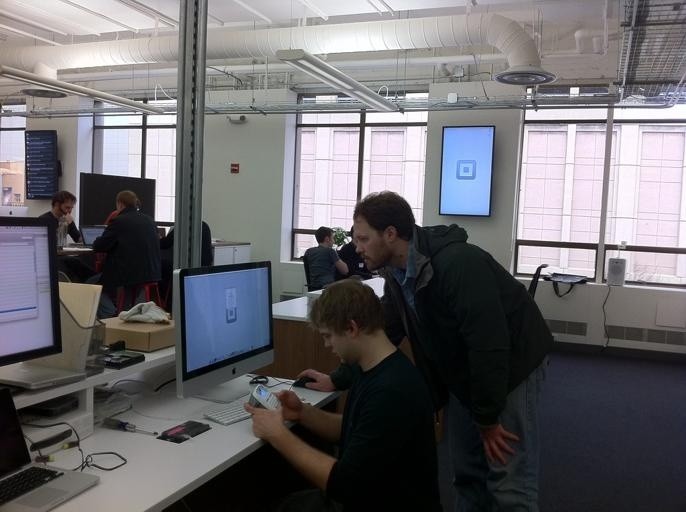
[214,246,250,266]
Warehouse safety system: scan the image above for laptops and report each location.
[78,225,109,249]
[0,364,87,389]
[0,382,101,511]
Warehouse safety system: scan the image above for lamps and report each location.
[276,49,397,112]
[1,66,163,115]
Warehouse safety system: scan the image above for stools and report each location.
[118,282,162,315]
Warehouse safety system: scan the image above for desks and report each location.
[12,346,342,511]
[272,275,385,380]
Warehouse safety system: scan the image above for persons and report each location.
[339,226,372,280]
[102,199,142,226]
[86,189,161,318]
[38,191,83,253]
[304,227,349,291]
[294,191,555,512]
[244,279,441,512]
[160,215,215,270]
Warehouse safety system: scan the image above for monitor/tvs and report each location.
[437,124,496,218]
[172,261,275,405]
[79,171,156,227]
[0,216,63,368]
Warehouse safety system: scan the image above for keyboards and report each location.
[203,403,254,427]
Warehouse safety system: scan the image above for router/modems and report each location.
[31,396,80,418]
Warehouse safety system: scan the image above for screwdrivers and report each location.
[45,440,81,456]
[103,416,159,435]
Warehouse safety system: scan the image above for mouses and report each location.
[292,377,318,389]
